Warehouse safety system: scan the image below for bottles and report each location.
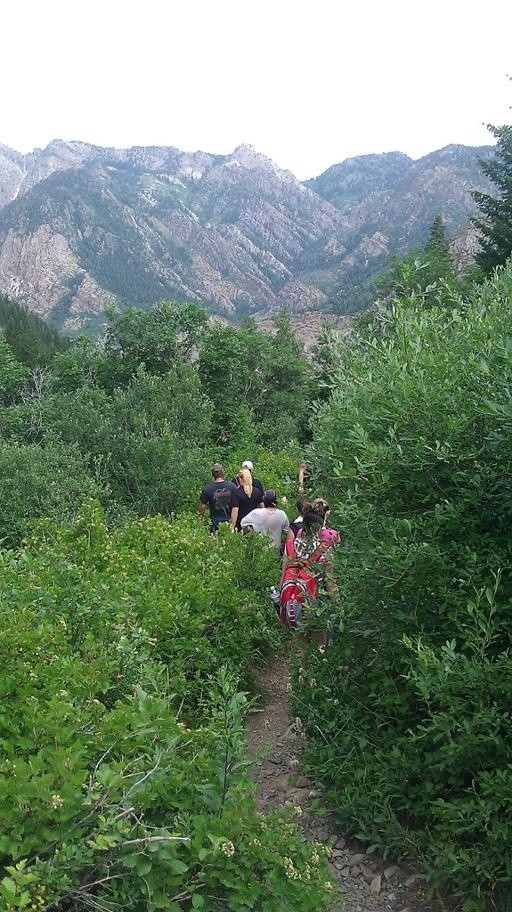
[270,586,281,614]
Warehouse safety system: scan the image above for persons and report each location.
[199,460,342,670]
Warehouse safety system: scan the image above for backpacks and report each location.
[278,540,330,627]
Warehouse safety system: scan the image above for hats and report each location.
[263,490,275,504]
[212,464,223,477]
[242,461,253,470]
